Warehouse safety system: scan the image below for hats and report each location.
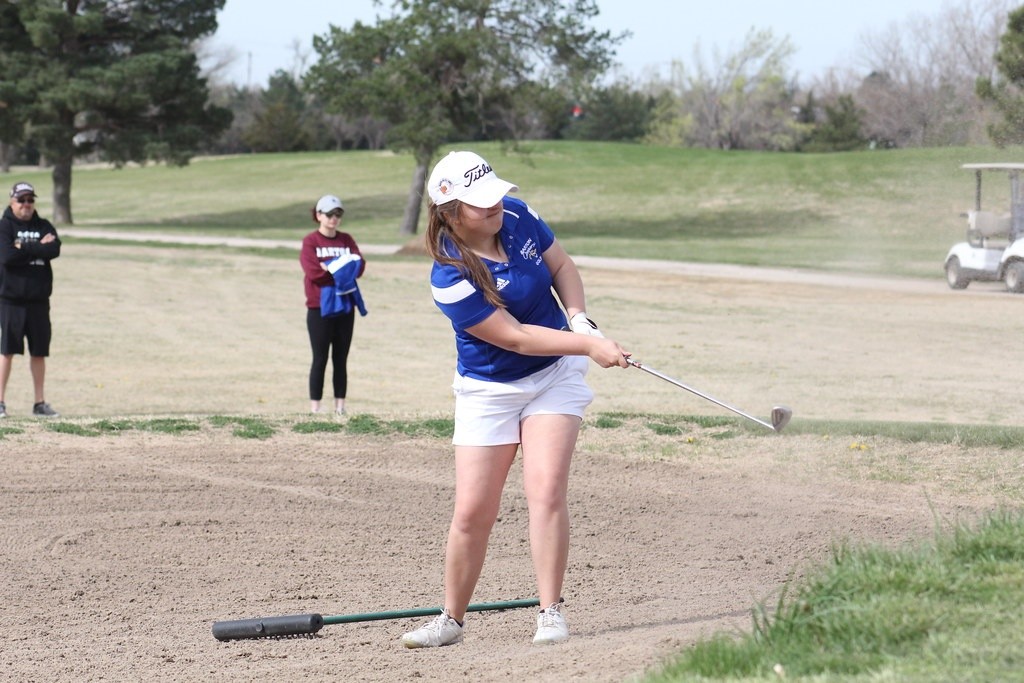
[9,181,38,199]
[316,194,344,214]
[426,150,520,210]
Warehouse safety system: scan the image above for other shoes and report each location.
[309,407,349,417]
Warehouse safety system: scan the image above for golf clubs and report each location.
[624,354,792,432]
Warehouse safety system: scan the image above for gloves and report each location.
[569,312,604,338]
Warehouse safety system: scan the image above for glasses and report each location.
[323,211,343,219]
[15,198,35,204]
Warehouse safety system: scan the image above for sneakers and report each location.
[0,402,8,418]
[400,613,468,649]
[532,604,571,645]
[33,402,59,418]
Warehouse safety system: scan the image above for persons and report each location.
[0,182,59,418]
[300,194,366,416]
[401,149,632,649]
